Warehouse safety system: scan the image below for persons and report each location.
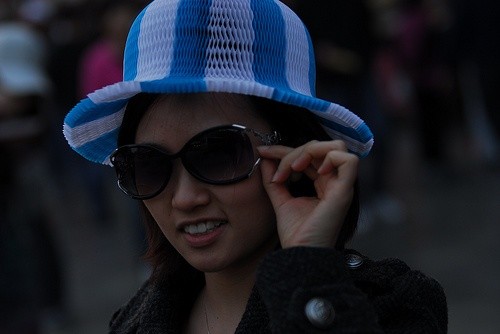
[62,0,448,334]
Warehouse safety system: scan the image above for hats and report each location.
[63,0,374,165]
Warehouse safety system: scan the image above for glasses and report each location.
[107,123,288,200]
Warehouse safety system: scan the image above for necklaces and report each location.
[202,307,210,334]
